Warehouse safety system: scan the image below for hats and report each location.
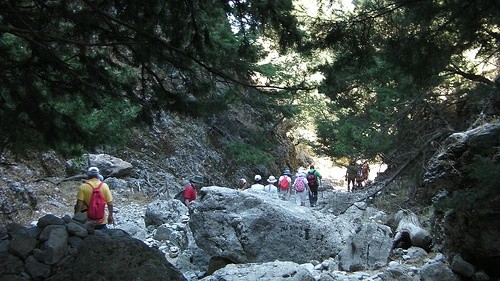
[88,166,100,173]
[296,171,304,176]
[284,170,291,174]
[267,176,277,182]
[192,176,205,184]
[255,175,262,180]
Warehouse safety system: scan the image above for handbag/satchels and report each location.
[174,190,185,204]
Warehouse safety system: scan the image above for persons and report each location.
[265,176,277,194]
[251,175,265,192]
[180,175,203,207]
[278,169,292,195]
[292,171,313,207]
[238,178,247,191]
[305,165,322,207]
[75,167,113,231]
[345,162,370,192]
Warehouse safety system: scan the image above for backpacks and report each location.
[280,176,289,189]
[307,170,317,186]
[295,178,305,191]
[84,182,105,219]
